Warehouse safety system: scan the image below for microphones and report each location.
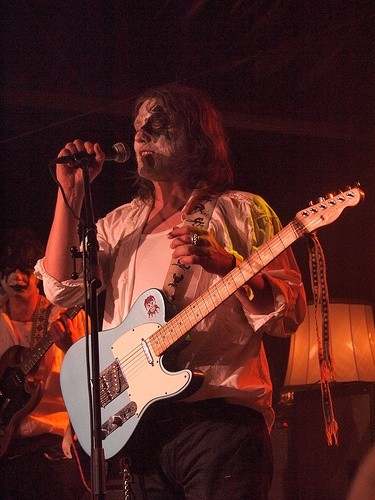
[53,142,131,164]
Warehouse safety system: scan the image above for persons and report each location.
[0,227,93,500]
[34,83,305,500]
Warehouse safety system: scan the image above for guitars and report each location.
[0,294,93,458]
[59,181,367,464]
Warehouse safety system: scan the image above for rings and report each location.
[190,231,201,246]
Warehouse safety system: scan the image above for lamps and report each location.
[282,298,375,390]
